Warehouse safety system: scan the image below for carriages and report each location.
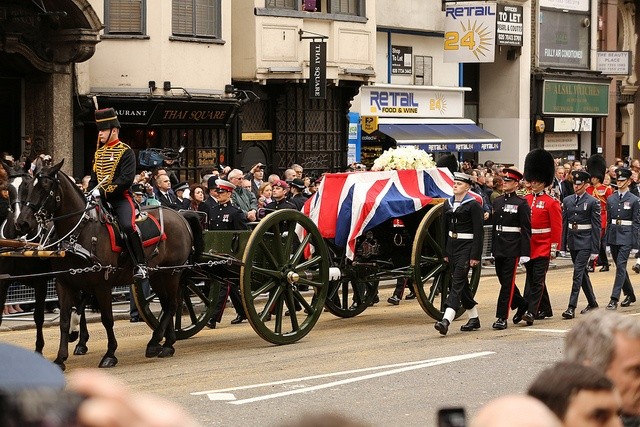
[0,155,483,372]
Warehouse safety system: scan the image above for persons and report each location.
[465,168,491,266]
[227,168,258,230]
[304,177,341,313]
[129,184,155,206]
[484,176,493,199]
[615,161,624,168]
[187,184,206,211]
[571,160,582,171]
[609,165,618,190]
[602,173,611,187]
[563,164,571,178]
[86,108,150,281]
[170,181,196,211]
[302,176,315,199]
[258,182,277,218]
[288,178,309,212]
[198,176,219,221]
[349,279,380,312]
[522,148,562,325]
[528,361,625,427]
[629,159,640,184]
[474,169,495,265]
[265,180,299,233]
[490,176,503,205]
[152,166,177,198]
[434,172,484,335]
[387,277,416,305]
[209,179,251,230]
[491,168,531,330]
[82,176,91,192]
[564,308,640,427]
[560,171,602,319]
[154,174,176,207]
[552,166,573,257]
[469,393,564,427]
[605,169,640,310]
[63,368,201,427]
[245,162,265,198]
[242,180,251,192]
[201,167,219,198]
[268,174,280,187]
[291,164,303,180]
[133,170,152,186]
[585,155,613,272]
[284,169,297,185]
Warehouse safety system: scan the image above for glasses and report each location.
[195,190,204,193]
[236,178,241,180]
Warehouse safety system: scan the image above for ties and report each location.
[620,194,622,200]
[576,196,580,203]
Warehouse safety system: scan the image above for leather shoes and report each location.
[599,265,609,271]
[621,295,636,306]
[435,318,450,334]
[405,291,416,299]
[285,305,301,315]
[537,308,552,318]
[632,263,640,272]
[522,312,534,325]
[513,299,528,323]
[138,317,145,321]
[607,299,618,308]
[304,308,309,313]
[581,302,598,313]
[324,308,329,311]
[258,312,271,320]
[493,318,507,328]
[130,317,138,321]
[461,317,480,330]
[349,300,360,310]
[374,296,379,303]
[388,295,399,304]
[562,306,575,318]
[207,317,216,328]
[231,314,247,324]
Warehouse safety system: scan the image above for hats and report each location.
[94,107,121,143]
[524,149,555,187]
[453,171,471,184]
[315,177,323,187]
[272,180,287,187]
[206,176,218,193]
[572,170,590,180]
[132,184,144,195]
[174,182,191,192]
[587,154,606,184]
[289,179,306,189]
[214,180,236,192]
[503,168,523,181]
[615,168,632,180]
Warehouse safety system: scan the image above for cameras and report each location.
[260,164,267,169]
[145,172,151,179]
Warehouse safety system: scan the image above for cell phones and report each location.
[437,407,466,427]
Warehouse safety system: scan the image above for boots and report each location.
[127,229,146,277]
